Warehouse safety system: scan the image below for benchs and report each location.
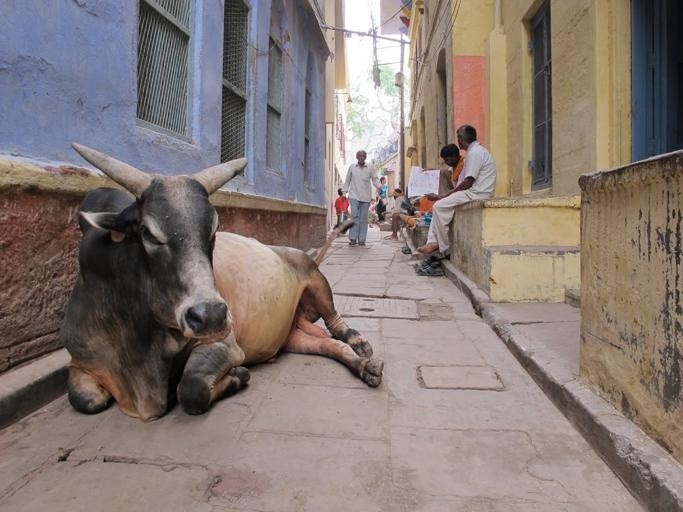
[409,224,429,248]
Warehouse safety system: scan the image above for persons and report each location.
[334,188,349,234]
[375,177,435,240]
[342,151,382,246]
[440,143,465,189]
[418,124,497,254]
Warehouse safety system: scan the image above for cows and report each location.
[58,143,385,421]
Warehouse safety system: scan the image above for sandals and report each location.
[402,247,450,276]
